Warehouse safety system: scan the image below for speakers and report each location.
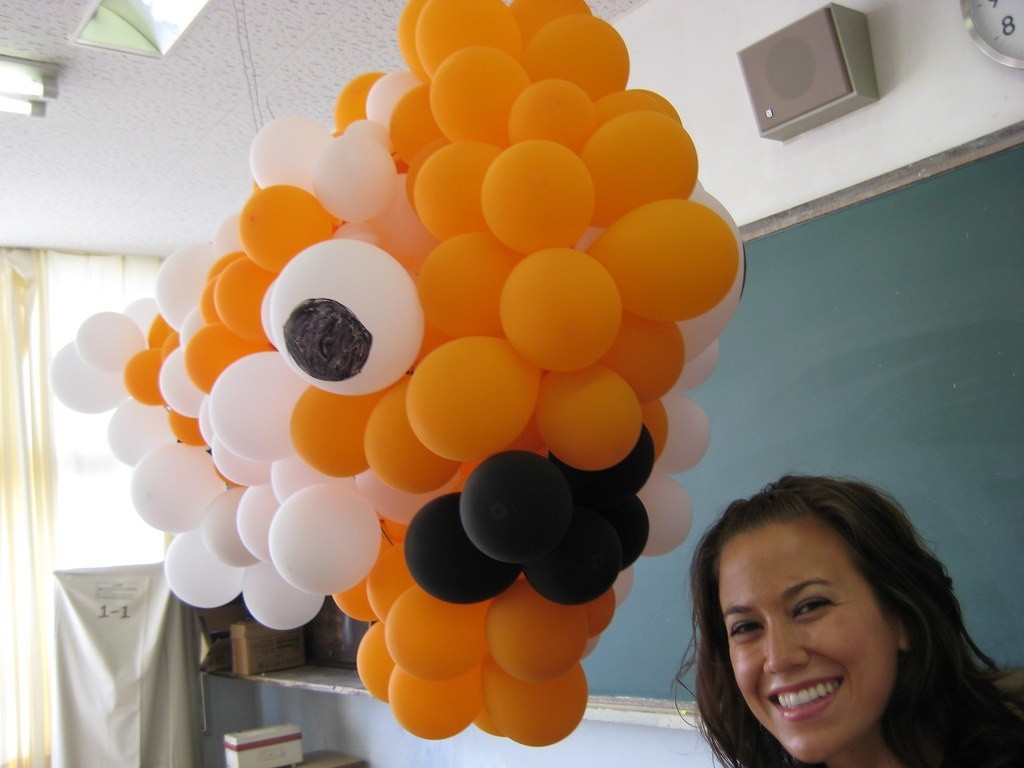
[736,2,880,142]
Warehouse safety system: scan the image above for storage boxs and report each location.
[231,622,306,675]
[224,723,304,768]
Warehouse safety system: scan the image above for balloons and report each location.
[51,1,745,747]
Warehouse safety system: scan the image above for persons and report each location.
[675,474,1024,768]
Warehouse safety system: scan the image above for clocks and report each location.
[961,0,1024,71]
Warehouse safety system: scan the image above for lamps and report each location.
[0,53,62,119]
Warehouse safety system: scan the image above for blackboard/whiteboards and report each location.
[582,123,1024,733]
